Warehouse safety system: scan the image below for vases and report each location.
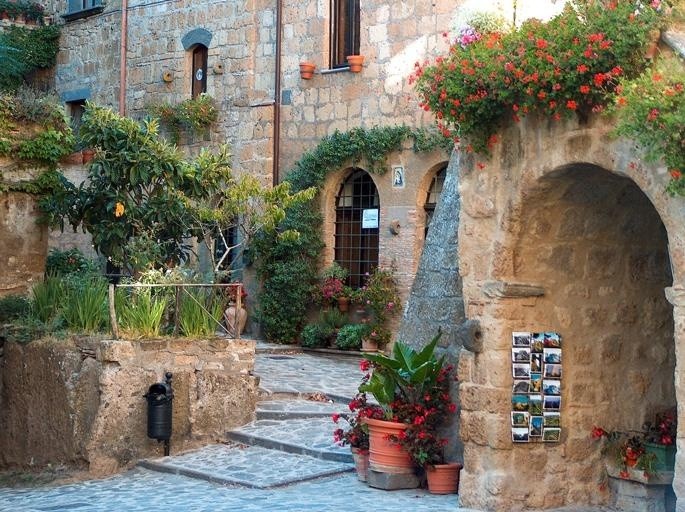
[223,303,248,335]
[425,461,463,495]
[298,61,318,80]
[347,55,364,74]
[642,438,677,472]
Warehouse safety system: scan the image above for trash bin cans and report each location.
[146,382,174,441]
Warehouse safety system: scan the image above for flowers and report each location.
[310,262,403,346]
[224,278,248,303]
[331,332,459,489]
[589,407,677,492]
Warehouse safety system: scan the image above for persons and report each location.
[395,171,402,185]
